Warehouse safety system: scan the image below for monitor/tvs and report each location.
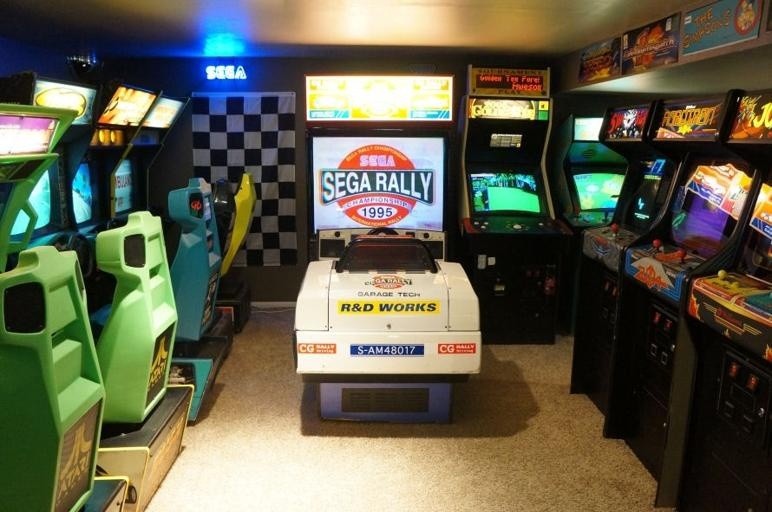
[312,136,444,234]
[634,175,662,220]
[747,183,772,284]
[470,172,540,213]
[72,163,93,224]
[10,169,51,236]
[573,173,625,210]
[114,159,132,213]
[671,163,753,257]
